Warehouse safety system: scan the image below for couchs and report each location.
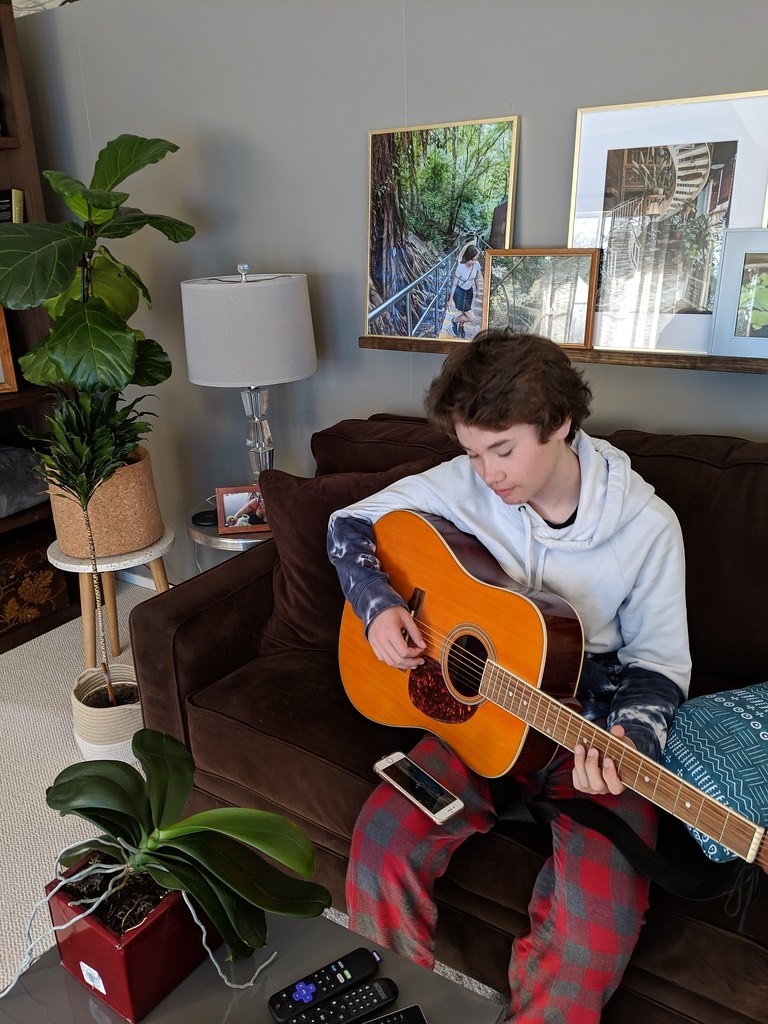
[128,413,768,1023]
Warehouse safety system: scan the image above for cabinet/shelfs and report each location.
[0,0,105,655]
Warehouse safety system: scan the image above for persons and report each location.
[447,245,482,337]
[227,492,267,525]
[327,326,692,1024]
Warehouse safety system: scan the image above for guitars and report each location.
[334,506,768,877]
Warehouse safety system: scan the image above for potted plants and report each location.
[17,364,161,765]
[46,728,332,1024]
[0,134,196,560]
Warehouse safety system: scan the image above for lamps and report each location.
[180,263,318,485]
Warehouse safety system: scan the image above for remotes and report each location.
[268,947,428,1024]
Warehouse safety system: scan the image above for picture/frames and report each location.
[567,91,768,360]
[0,303,18,394]
[708,228,768,359]
[364,116,521,343]
[480,248,601,350]
[215,486,272,534]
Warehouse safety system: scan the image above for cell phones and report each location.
[373,752,464,825]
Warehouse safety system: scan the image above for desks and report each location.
[46,526,174,670]
[0,907,506,1023]
[186,498,274,573]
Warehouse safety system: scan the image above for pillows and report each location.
[258,458,443,656]
[661,679,768,863]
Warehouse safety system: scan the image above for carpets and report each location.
[0,581,161,992]
